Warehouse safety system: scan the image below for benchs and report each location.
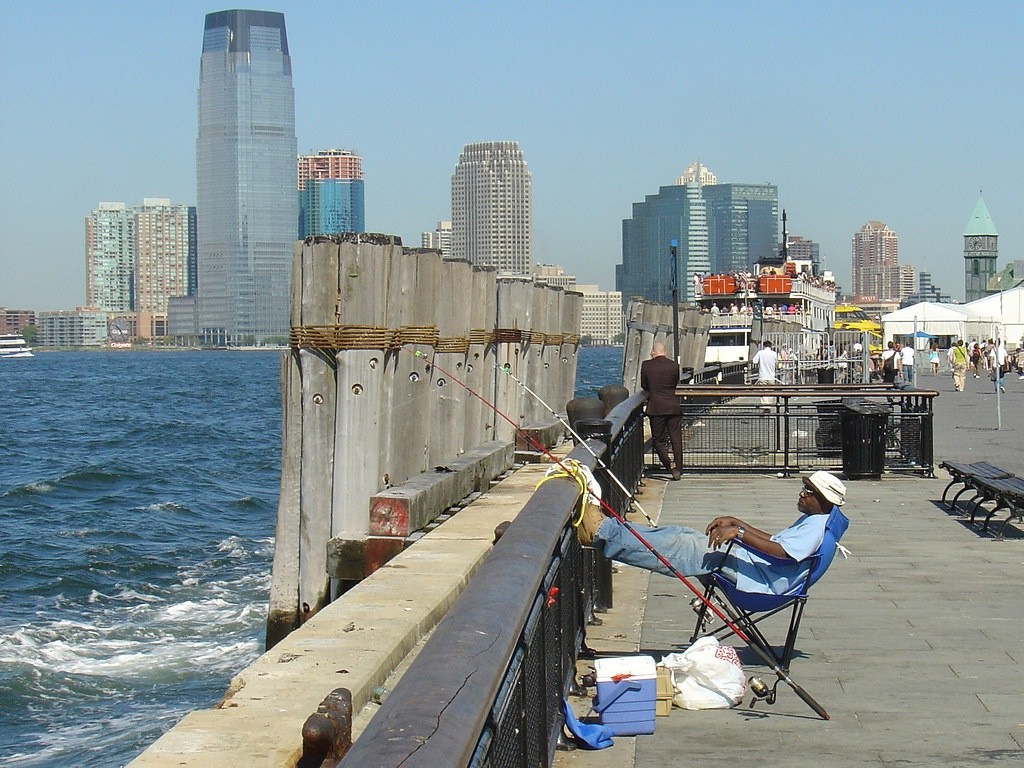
[938,457,1024,545]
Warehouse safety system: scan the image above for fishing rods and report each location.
[492,363,773,661]
[398,344,832,721]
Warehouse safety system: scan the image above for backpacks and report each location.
[972,353,980,365]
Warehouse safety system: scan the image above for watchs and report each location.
[737,527,746,540]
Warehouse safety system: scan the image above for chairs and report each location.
[689,503,851,680]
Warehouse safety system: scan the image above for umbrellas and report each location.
[902,331,939,347]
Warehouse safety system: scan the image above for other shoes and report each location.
[667,462,681,481]
[974,373,976,377]
[1000,386,1006,393]
[1019,376,1024,380]
[976,376,980,378]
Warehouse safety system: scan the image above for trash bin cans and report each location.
[842,396,890,480]
[817,367,834,384]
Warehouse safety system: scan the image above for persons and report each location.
[880,341,902,402]
[696,301,800,328]
[946,343,957,372]
[577,471,847,596]
[929,343,940,374]
[640,343,684,481]
[901,342,914,384]
[771,343,798,384]
[693,267,837,291]
[753,341,776,416]
[816,340,875,383]
[963,339,1024,393]
[952,340,967,391]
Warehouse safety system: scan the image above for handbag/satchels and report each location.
[989,365,1004,381]
[657,636,746,709]
[884,351,897,374]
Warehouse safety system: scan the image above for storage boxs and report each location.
[593,654,661,738]
[651,665,676,719]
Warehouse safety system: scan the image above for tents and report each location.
[881,287,1024,350]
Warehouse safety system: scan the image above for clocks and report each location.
[968,236,983,250]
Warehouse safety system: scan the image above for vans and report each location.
[833,304,881,335]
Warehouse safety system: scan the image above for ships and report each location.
[676,209,837,372]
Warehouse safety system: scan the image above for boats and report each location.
[0,334,33,355]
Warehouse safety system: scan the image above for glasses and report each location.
[801,486,813,494]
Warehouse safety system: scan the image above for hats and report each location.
[803,470,847,506]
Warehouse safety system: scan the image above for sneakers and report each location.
[575,493,608,546]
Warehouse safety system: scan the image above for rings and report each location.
[716,536,720,540]
[720,538,724,541]
